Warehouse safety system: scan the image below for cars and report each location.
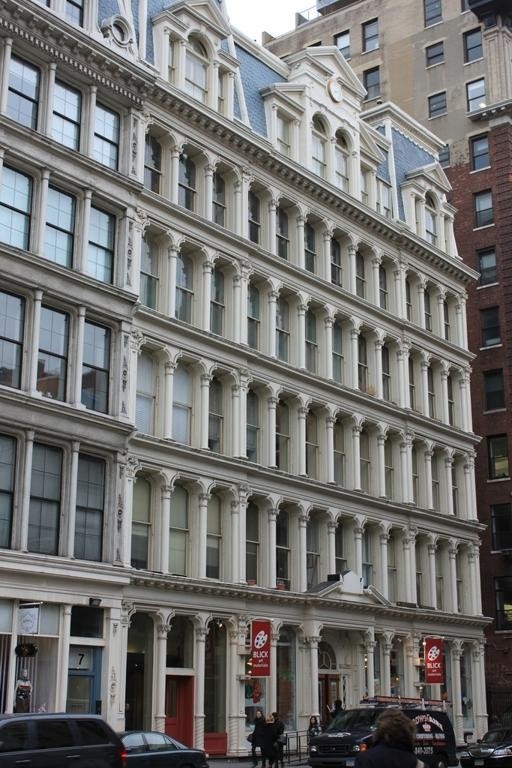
[458,725,512,767]
[115,727,210,767]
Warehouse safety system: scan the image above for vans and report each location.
[0,710,128,766]
[305,693,460,767]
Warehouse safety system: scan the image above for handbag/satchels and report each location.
[246,733,256,743]
[276,733,287,745]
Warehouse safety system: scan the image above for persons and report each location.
[327,699,344,718]
[267,712,276,767]
[252,710,266,767]
[354,707,431,767]
[271,712,285,767]
[12,669,32,713]
[306,713,319,753]
